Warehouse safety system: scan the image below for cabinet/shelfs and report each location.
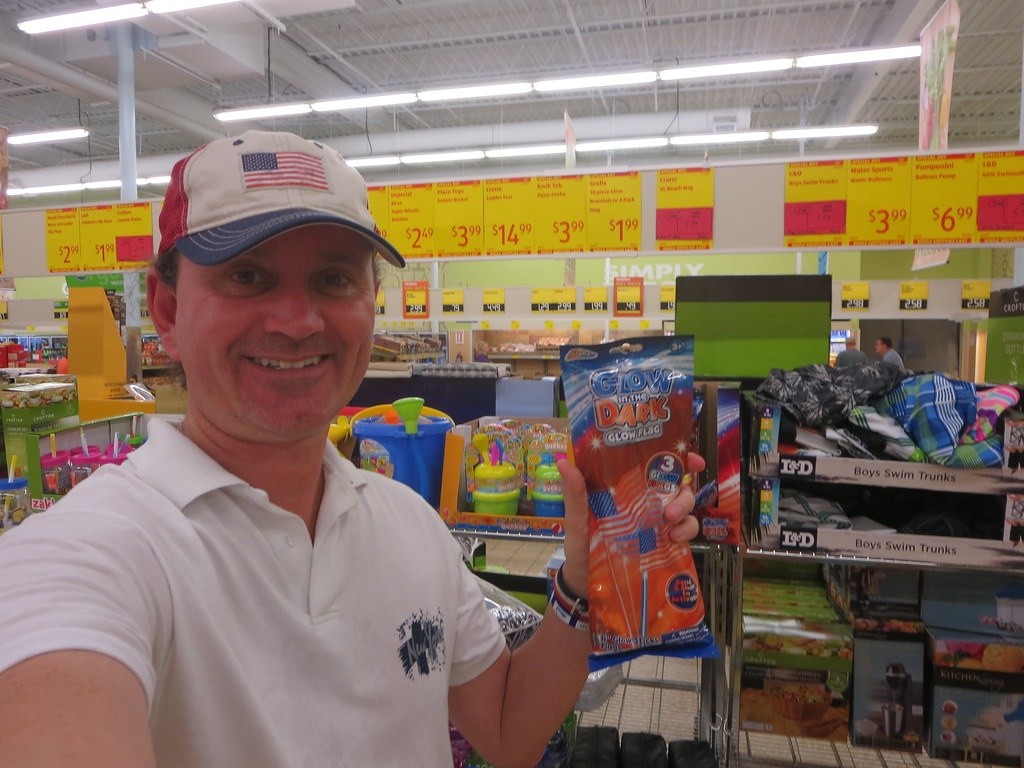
[449,525,728,768]
[731,547,1024,768]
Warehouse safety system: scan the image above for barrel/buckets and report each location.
[352,418,450,509]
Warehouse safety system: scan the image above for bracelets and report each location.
[554,565,596,623]
[550,591,590,631]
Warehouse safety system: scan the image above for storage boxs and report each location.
[738,459,1024,565]
[738,389,1024,493]
[738,565,1024,767]
[24,410,185,513]
[439,416,566,539]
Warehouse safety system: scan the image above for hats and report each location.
[159,130,407,268]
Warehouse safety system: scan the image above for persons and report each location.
[0,129,705,767]
[876,336,904,370]
[835,338,868,369]
